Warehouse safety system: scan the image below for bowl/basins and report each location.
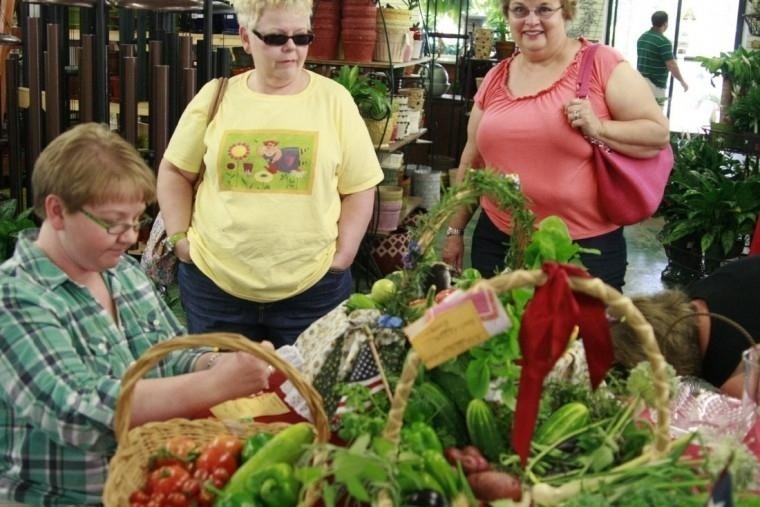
[659,381,755,447]
[421,81,452,97]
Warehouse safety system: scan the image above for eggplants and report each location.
[406,490,441,507]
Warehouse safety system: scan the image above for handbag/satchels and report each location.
[590,135,675,228]
[140,210,177,289]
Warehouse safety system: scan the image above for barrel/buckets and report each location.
[413,170,441,209]
[376,186,404,231]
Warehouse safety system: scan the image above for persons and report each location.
[0,121,275,507]
[637,10,689,107]
[611,256,760,399]
[157,0,385,348]
[443,0,671,294]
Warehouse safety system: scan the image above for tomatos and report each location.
[130,437,241,507]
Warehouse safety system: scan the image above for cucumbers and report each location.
[466,399,507,466]
[231,422,315,485]
[419,384,468,444]
[532,401,589,458]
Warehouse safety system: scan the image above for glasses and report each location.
[79,207,153,235]
[507,4,562,20]
[253,29,316,46]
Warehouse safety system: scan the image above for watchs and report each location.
[165,232,187,253]
[446,227,464,235]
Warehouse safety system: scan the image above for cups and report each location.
[472,25,492,60]
[741,348,759,409]
[365,87,443,235]
[308,1,423,63]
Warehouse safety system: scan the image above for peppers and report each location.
[244,462,301,507]
[241,433,273,462]
[407,451,456,498]
[204,480,266,507]
[402,422,443,456]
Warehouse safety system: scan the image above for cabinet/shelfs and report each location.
[298,56,436,292]
[457,57,500,169]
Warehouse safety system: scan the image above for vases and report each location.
[472,29,494,60]
[311,0,441,276]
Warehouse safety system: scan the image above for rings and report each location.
[574,111,580,119]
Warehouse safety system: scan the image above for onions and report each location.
[469,471,521,501]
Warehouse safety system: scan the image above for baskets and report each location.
[100,332,332,506]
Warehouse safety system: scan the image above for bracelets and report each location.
[207,352,222,368]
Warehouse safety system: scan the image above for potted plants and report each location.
[492,9,516,62]
[653,45,760,287]
